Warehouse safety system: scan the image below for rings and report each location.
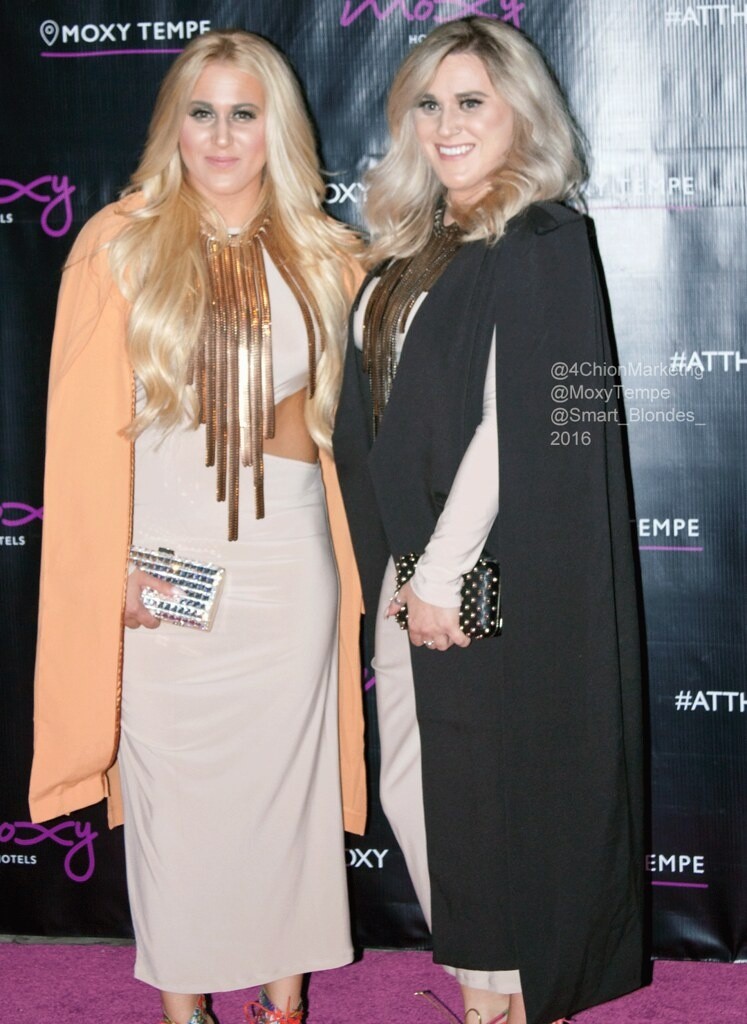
[392,596,405,606]
[422,640,435,646]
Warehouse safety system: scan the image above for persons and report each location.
[350,16,662,1024]
[29,30,360,1024]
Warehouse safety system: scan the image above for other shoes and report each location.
[243,988,304,1023]
[159,994,217,1024]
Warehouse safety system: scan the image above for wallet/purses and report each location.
[394,553,504,640]
[127,545,225,633]
